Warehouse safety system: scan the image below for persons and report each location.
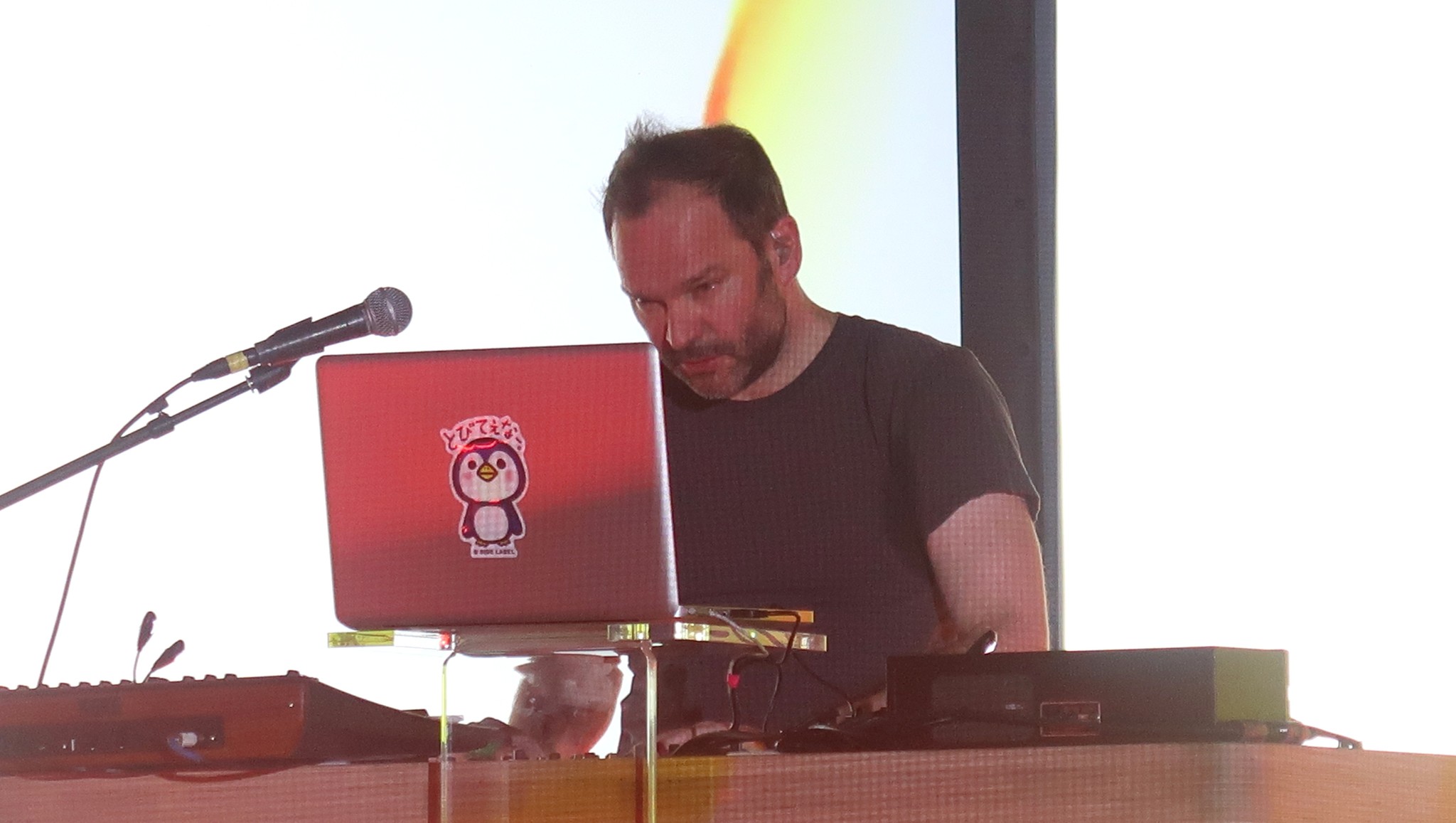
[465,126,1049,759]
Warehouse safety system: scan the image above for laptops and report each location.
[314,342,816,632]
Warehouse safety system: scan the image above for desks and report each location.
[0,739,1456,823]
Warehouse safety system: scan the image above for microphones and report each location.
[142,639,185,683]
[132,611,156,683]
[189,284,414,381]
